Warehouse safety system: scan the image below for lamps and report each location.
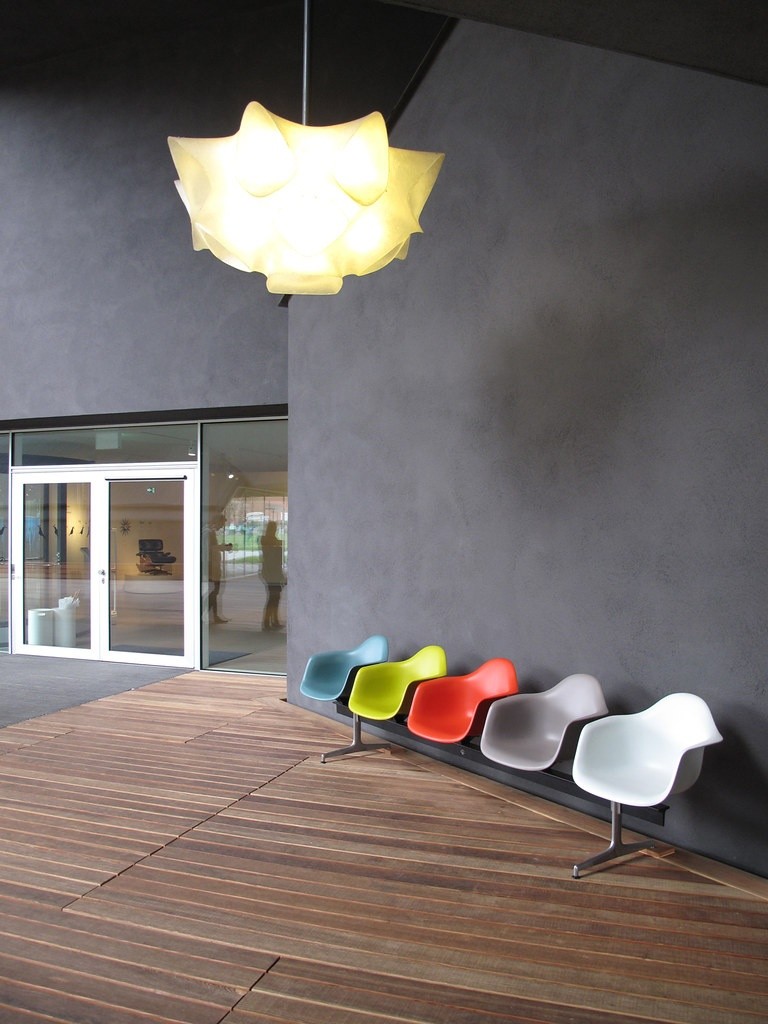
[166,0,447,296]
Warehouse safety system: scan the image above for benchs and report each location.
[299,633,724,881]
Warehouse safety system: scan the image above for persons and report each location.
[201,513,232,624]
[261,522,288,632]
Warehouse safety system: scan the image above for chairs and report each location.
[135,539,176,572]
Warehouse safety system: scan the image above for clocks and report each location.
[119,517,132,535]
